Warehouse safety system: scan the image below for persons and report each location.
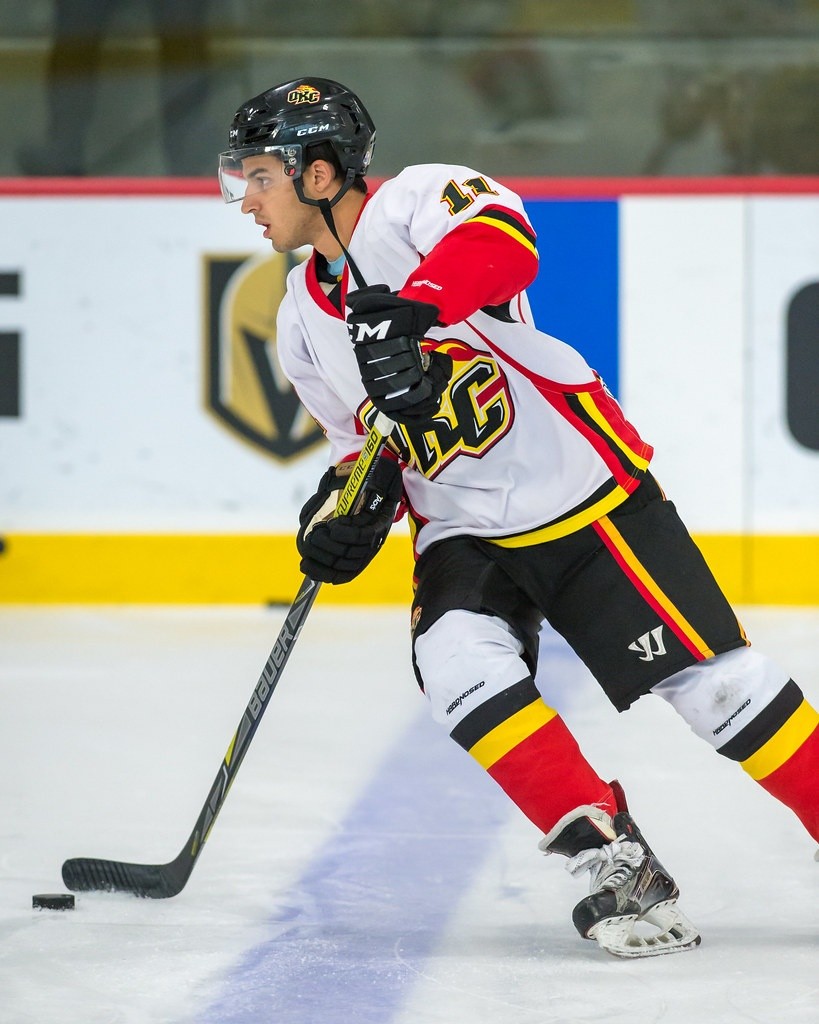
[219,77,818,957]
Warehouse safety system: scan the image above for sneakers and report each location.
[538,778,703,958]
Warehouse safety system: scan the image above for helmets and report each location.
[228,80,379,173]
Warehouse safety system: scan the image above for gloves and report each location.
[295,458,403,586]
[342,286,454,435]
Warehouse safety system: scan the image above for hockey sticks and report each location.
[61,409,396,899]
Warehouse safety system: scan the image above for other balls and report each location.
[31,894,75,910]
